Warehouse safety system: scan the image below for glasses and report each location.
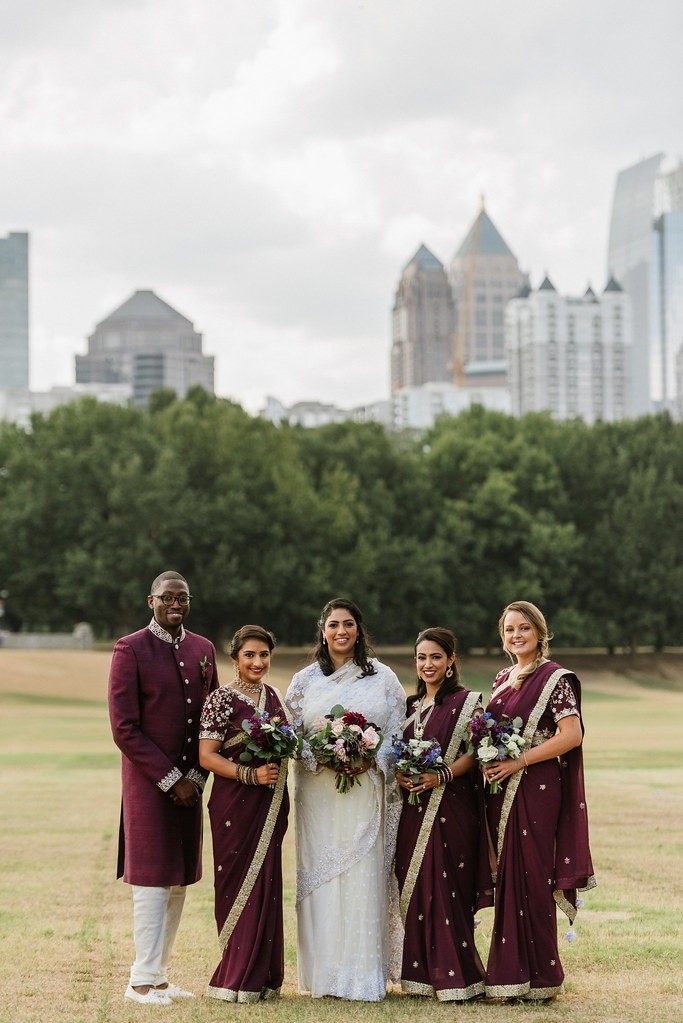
[150,595,193,607]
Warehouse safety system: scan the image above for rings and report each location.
[423,784,425,789]
[360,767,362,771]
[270,763,273,768]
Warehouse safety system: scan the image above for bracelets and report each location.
[236,764,259,786]
[522,752,529,775]
[438,766,453,786]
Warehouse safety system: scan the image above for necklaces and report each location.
[414,693,435,739]
[235,677,263,694]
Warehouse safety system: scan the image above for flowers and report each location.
[300,704,385,794]
[241,709,298,788]
[382,734,446,805]
[457,711,527,795]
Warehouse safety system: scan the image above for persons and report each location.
[199,625,296,1006]
[285,598,408,1003]
[108,570,222,1006]
[392,627,496,1004]
[482,600,597,1007]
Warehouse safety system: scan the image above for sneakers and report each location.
[125,986,173,1007]
[153,985,195,999]
